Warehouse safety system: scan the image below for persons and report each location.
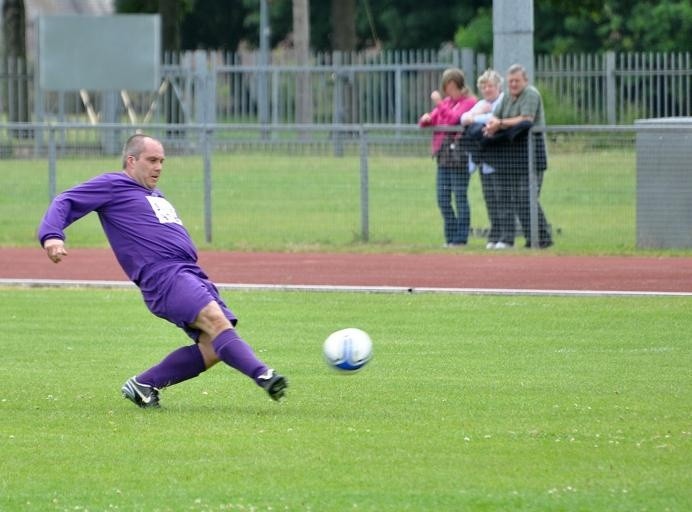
[36,135,289,409]
[418,64,555,249]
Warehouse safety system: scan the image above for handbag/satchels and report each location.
[438,137,469,167]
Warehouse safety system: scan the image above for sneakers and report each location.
[257,369,287,401]
[121,375,163,409]
[487,241,513,249]
